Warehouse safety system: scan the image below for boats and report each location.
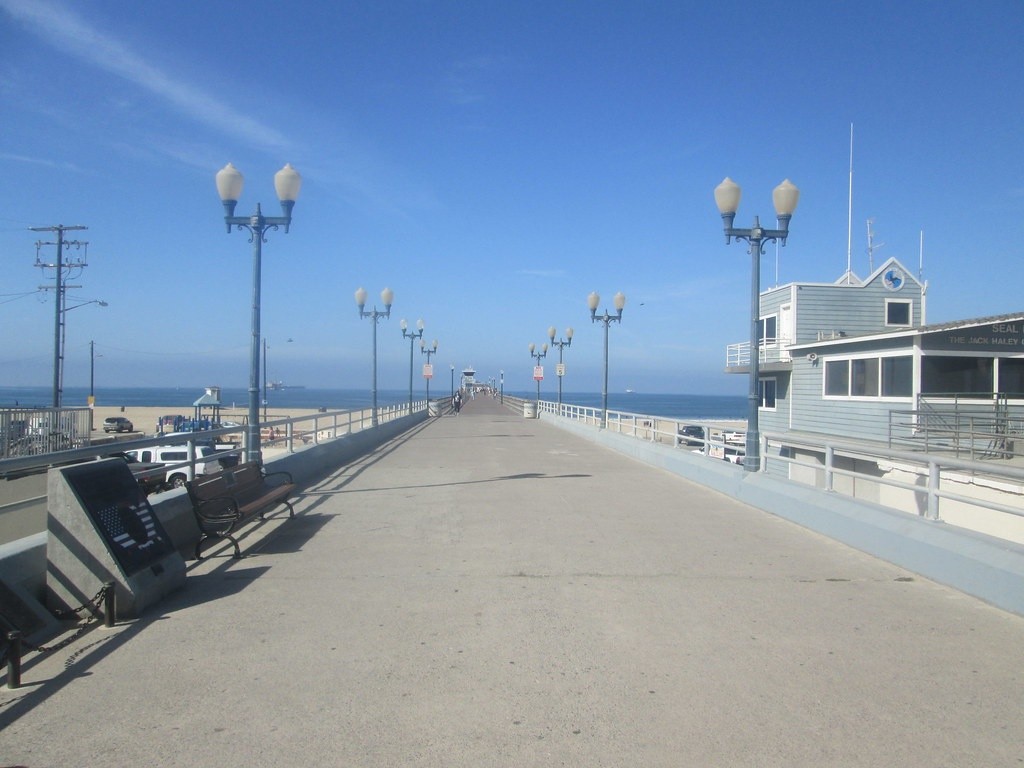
[266,376,288,392]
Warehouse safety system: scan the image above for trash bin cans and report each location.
[523,402,537,419]
[428,401,442,417]
[319,407,327,412]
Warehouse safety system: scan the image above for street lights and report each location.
[356,287,394,427]
[31,222,110,408]
[214,161,302,468]
[529,340,548,401]
[498,369,506,396]
[586,289,626,431]
[713,176,800,474]
[400,318,424,415]
[449,363,456,398]
[418,337,439,413]
[548,325,574,414]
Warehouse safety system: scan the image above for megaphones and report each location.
[807,352,817,361]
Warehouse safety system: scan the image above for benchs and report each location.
[185,461,297,560]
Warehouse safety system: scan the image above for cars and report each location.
[678,425,708,446]
[6,418,26,437]
[720,427,751,442]
[219,421,241,429]
[103,416,133,433]
[156,414,190,432]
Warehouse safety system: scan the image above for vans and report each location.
[27,416,79,437]
[116,445,222,491]
